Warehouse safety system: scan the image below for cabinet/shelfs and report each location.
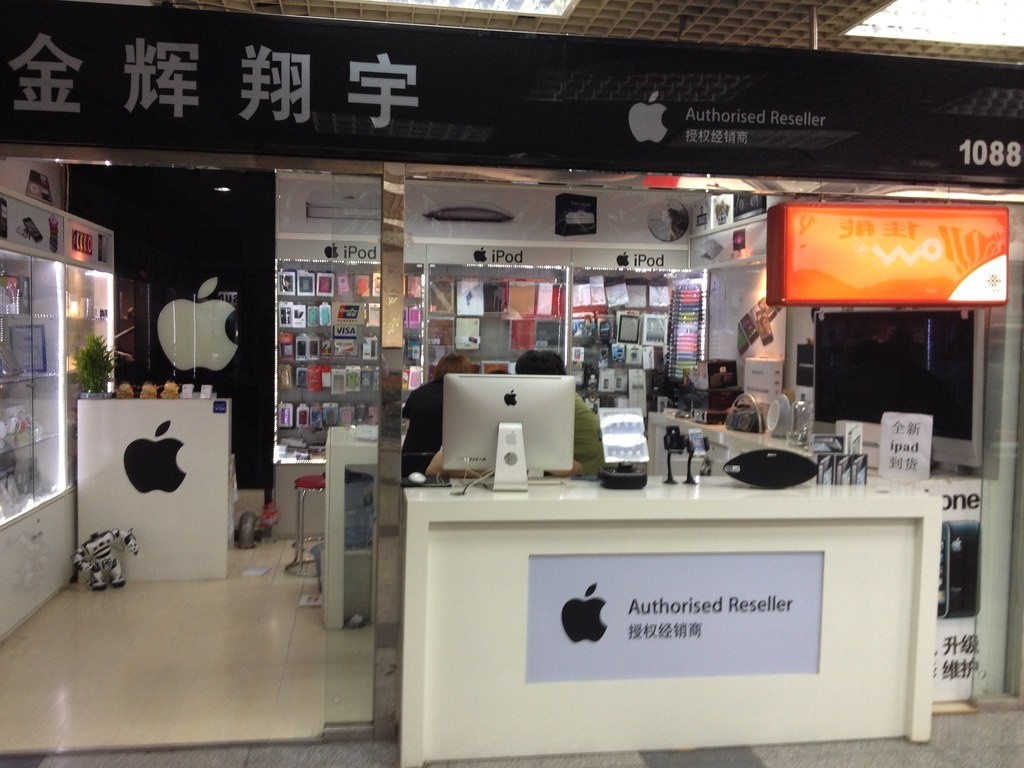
[1,189,116,643]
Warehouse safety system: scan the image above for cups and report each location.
[260,503,278,541]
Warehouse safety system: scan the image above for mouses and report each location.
[409,472,427,483]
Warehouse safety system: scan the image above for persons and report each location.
[425,370,509,476]
[515,351,618,476]
[401,352,472,478]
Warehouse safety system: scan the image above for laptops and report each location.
[700,238,723,259]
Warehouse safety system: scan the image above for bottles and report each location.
[788,394,809,447]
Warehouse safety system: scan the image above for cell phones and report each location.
[0,198,8,237]
[738,297,781,356]
[936,521,981,618]
[71,229,108,262]
[23,218,43,243]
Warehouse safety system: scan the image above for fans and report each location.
[645,196,689,242]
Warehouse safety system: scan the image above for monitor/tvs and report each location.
[733,228,746,251]
[813,310,973,440]
[441,373,575,491]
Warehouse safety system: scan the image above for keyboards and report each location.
[449,476,563,488]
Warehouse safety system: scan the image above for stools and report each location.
[284,473,325,576]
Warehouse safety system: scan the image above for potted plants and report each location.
[71,331,119,399]
